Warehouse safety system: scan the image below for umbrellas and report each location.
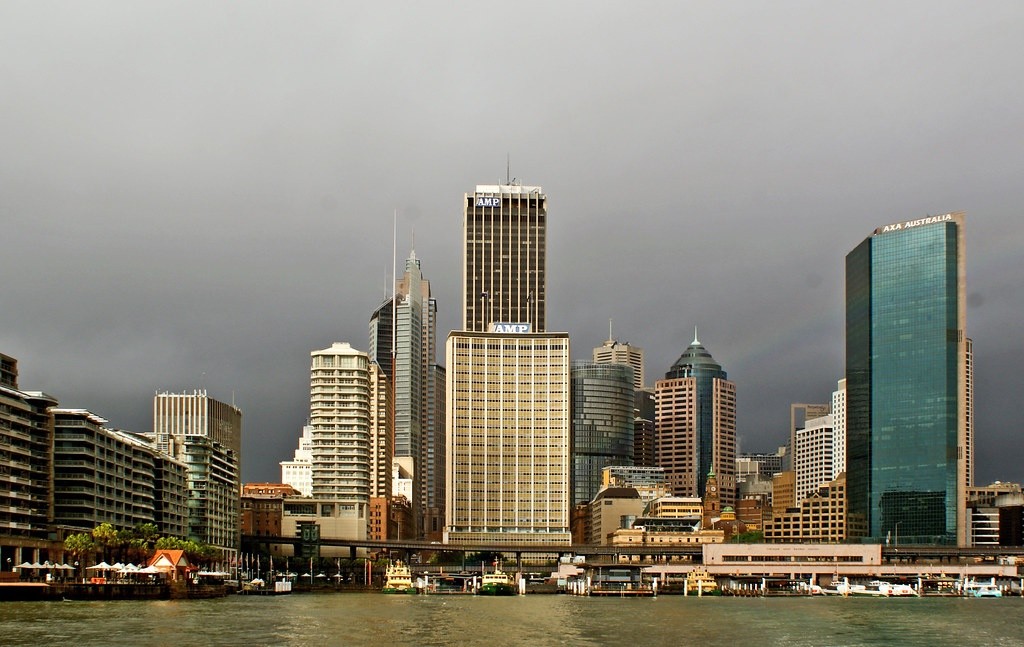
[276,573,343,577]
[85,562,159,583]
[14,562,76,582]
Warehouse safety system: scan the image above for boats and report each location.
[683,566,722,596]
[478,556,518,595]
[382,558,423,595]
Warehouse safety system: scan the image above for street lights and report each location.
[6,558,12,582]
[74,561,79,584]
[894,521,903,555]
